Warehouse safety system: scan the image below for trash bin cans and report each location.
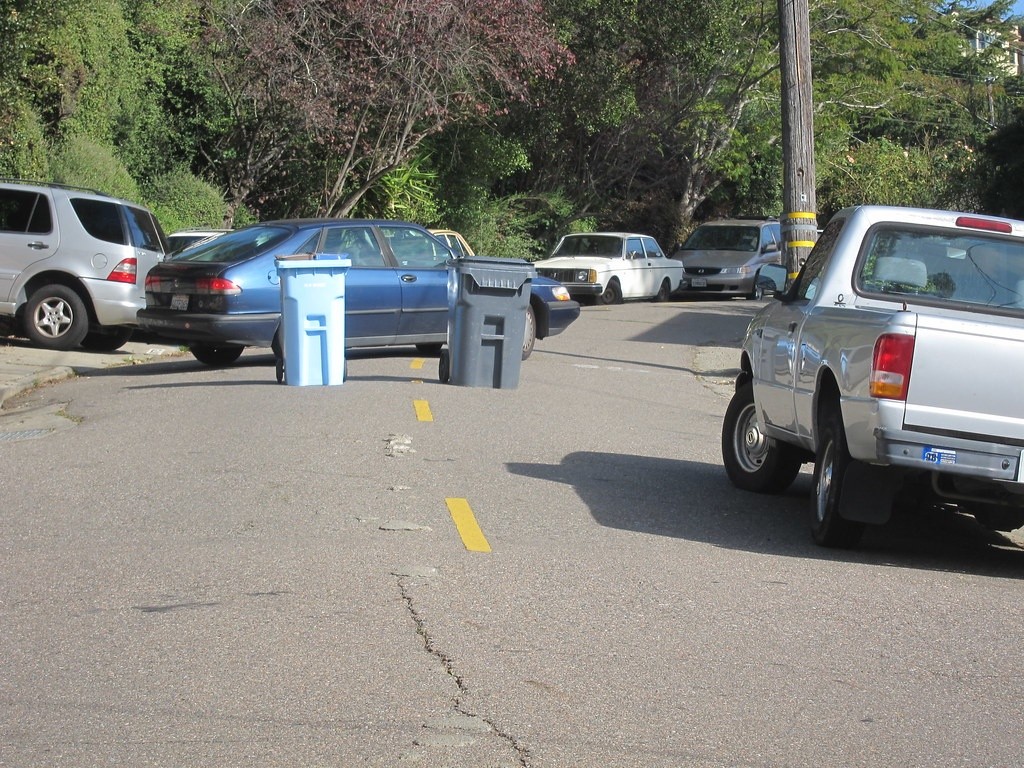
[270,250,352,387]
[438,255,540,390]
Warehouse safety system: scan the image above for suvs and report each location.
[0,173,170,353]
[670,218,784,302]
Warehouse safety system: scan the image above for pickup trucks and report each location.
[721,203,1024,551]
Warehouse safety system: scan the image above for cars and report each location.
[134,216,580,364]
[525,231,684,304]
[424,227,475,259]
[166,227,243,257]
